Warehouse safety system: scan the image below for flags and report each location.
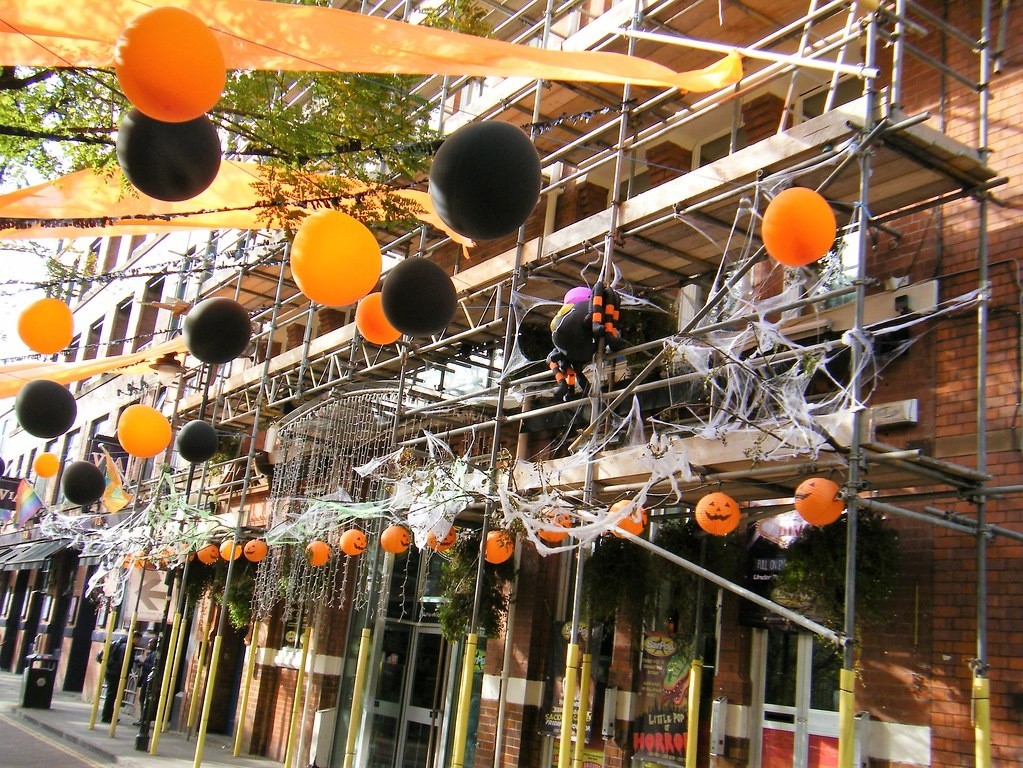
[12,477,45,530]
[102,453,135,514]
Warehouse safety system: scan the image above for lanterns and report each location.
[116,478,846,573]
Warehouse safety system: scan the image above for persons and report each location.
[100,630,143,722]
[132,636,166,726]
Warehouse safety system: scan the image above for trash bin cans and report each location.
[19,653,59,710]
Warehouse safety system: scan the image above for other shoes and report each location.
[101,716,121,724]
[132,719,142,725]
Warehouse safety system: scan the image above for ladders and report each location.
[218,279,511,430]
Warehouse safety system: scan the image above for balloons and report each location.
[117,404,174,458]
[283,211,385,307]
[117,99,222,201]
[355,289,403,346]
[424,118,543,237]
[16,297,76,356]
[17,379,78,442]
[383,256,456,337]
[174,419,219,463]
[180,298,254,364]
[111,8,229,124]
[61,460,106,507]
[758,185,841,266]
[33,451,60,476]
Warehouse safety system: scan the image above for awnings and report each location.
[0,537,72,573]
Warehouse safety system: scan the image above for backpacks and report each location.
[96,639,127,668]
